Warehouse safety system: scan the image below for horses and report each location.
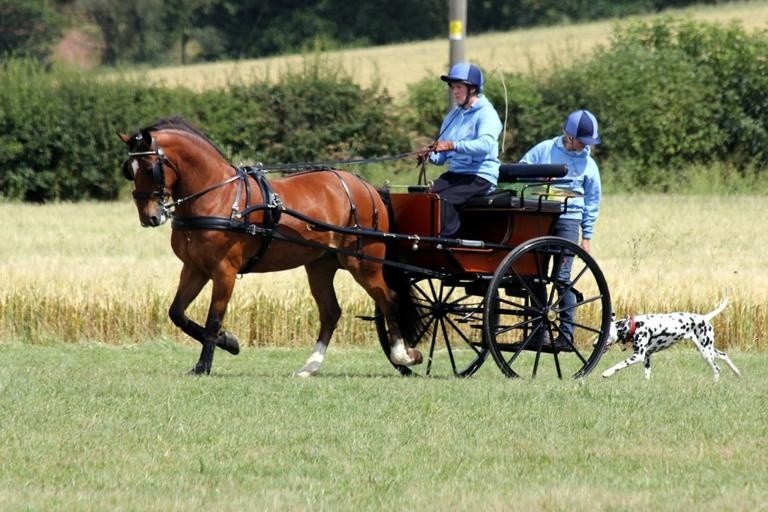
[117,115,437,380]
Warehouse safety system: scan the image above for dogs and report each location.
[592,295,741,384]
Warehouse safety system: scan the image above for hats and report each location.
[437,61,485,94]
[560,108,602,147]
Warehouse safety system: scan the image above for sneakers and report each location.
[511,330,572,350]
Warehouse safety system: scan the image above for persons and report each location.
[517,109,602,348]
[419,63,504,237]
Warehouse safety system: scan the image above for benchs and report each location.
[457,187,532,246]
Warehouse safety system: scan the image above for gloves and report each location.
[431,139,455,154]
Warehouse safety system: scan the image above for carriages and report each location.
[110,113,619,385]
